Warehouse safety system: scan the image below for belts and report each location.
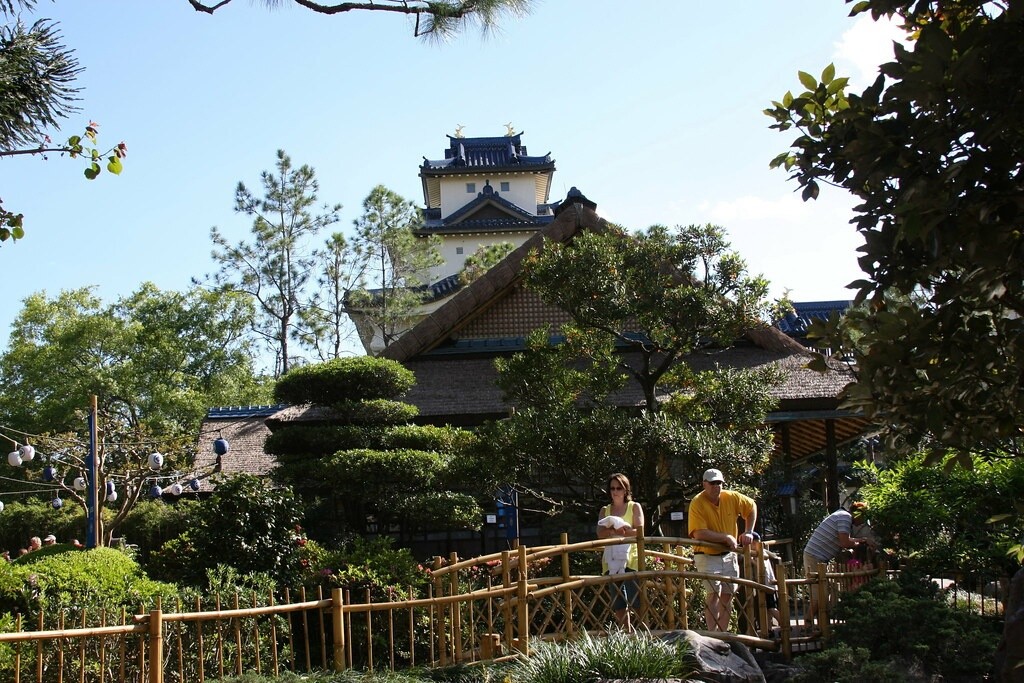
[693,550,731,556]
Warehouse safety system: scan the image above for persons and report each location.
[72,539,80,546]
[802,502,867,634]
[44,535,56,546]
[597,473,644,632]
[737,532,793,638]
[688,468,758,633]
[2,537,42,561]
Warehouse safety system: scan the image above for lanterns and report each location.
[44,466,56,480]
[172,484,182,495]
[52,498,62,509]
[74,476,87,490]
[190,478,200,491]
[107,478,118,503]
[148,450,163,470]
[8,451,22,467]
[213,438,228,455]
[151,485,162,497]
[19,445,35,463]
[0,501,4,512]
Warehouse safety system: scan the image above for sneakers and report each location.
[799,627,816,637]
[756,624,781,635]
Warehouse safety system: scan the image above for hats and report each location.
[855,502,869,514]
[703,469,726,482]
[753,532,761,540]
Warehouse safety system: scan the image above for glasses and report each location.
[611,486,623,491]
[710,480,721,485]
